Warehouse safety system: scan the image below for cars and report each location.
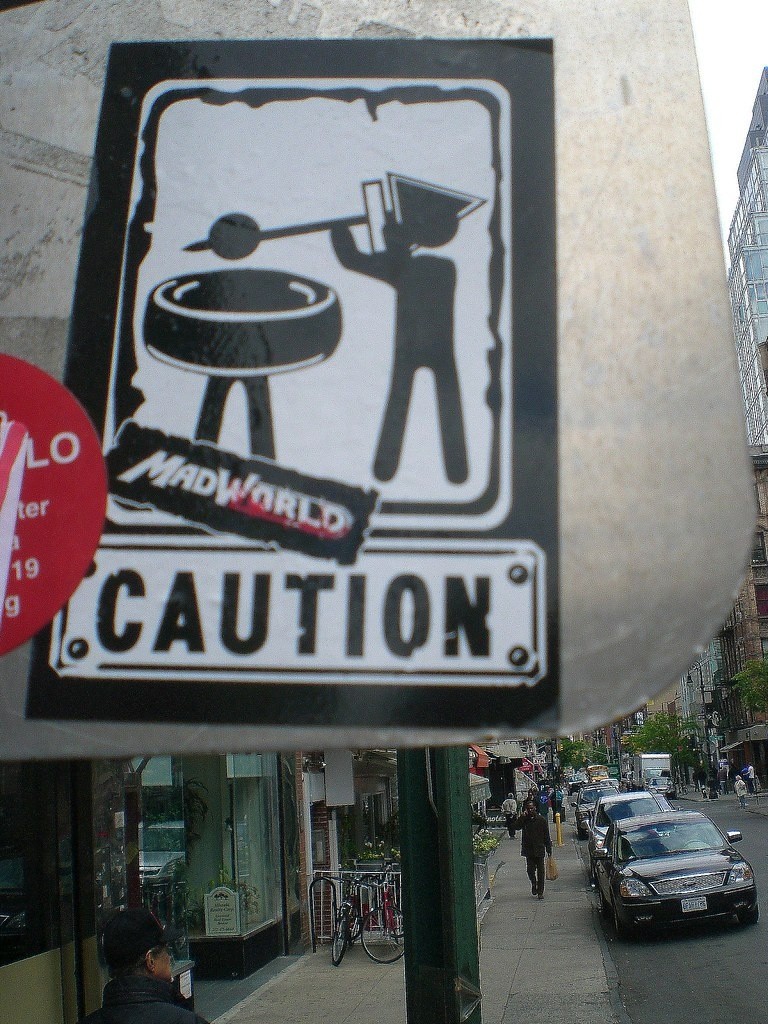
[579,790,684,887]
[591,807,759,941]
[641,776,677,800]
[570,785,627,840]
[566,759,634,797]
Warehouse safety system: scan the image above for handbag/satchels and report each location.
[546,856,559,880]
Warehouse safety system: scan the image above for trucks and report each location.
[633,752,673,792]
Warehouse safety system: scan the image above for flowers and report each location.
[472,829,500,855]
[357,840,399,859]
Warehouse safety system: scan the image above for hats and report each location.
[103,906,185,965]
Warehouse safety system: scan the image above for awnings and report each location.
[720,741,743,752]
[470,745,489,767]
[484,744,525,759]
[518,758,544,774]
[367,757,491,806]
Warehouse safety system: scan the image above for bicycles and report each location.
[322,861,405,966]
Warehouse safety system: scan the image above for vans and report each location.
[136,822,186,905]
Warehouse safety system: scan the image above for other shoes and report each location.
[538,893,544,899]
[532,883,537,895]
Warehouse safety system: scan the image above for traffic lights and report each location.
[559,743,563,752]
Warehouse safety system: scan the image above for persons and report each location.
[501,785,564,839]
[76,907,210,1024]
[734,775,747,810]
[692,763,754,795]
[511,801,553,899]
